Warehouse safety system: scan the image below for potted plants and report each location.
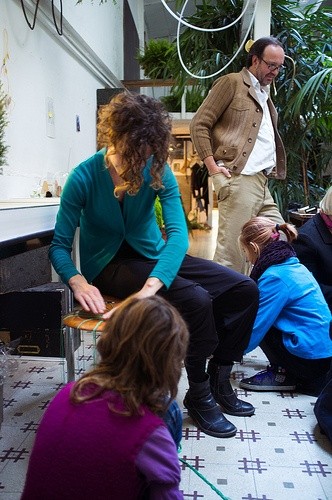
[271,0,332,227]
[136,38,175,80]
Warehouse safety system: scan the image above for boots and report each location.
[207,358,255,417]
[183,372,237,438]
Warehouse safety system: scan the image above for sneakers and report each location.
[238,362,297,391]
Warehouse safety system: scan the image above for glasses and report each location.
[259,55,286,74]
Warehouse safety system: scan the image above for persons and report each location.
[49,88,260,438]
[19,294,189,500]
[239,217,332,390]
[190,37,288,275]
[295,186,332,312]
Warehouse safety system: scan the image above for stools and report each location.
[64,294,122,385]
